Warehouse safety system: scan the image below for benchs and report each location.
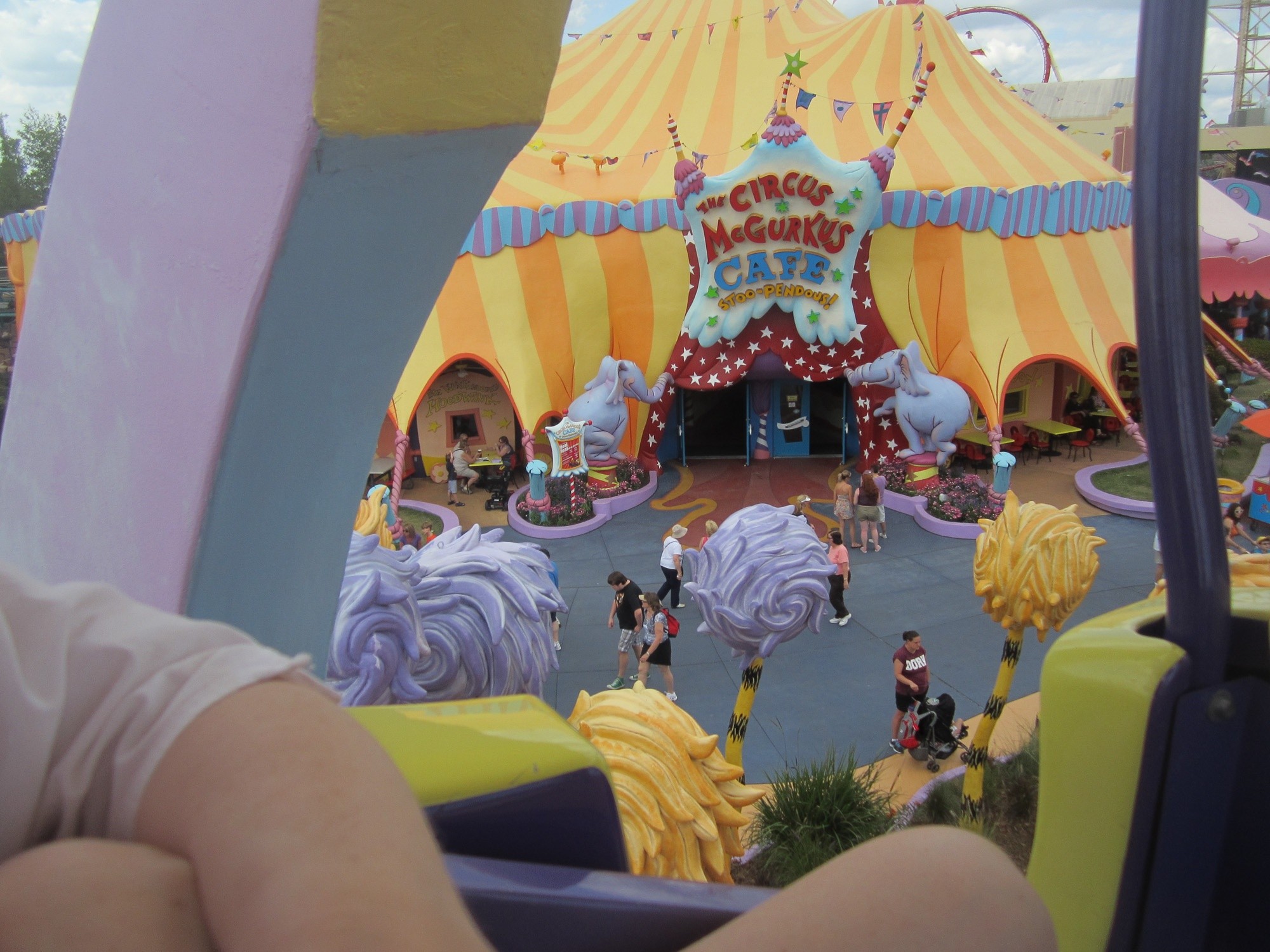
[384,468,416,492]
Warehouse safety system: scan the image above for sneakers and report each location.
[553,641,561,651]
[839,613,851,626]
[829,618,841,624]
[607,677,625,690]
[664,691,677,701]
[629,672,651,681]
[889,739,904,754]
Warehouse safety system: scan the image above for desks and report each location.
[369,459,395,487]
[1090,408,1131,442]
[469,451,503,489]
[955,432,1015,470]
[1023,418,1082,456]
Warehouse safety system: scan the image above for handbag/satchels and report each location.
[907,746,929,761]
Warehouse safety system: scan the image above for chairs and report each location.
[1027,431,1053,464]
[1006,432,1026,465]
[1102,416,1121,446]
[952,439,988,475]
[496,448,526,489]
[1068,428,1095,462]
[456,476,470,495]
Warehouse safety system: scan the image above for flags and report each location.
[1200,108,1270,178]
[794,89,815,109]
[971,48,1068,132]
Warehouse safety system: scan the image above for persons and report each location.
[699,520,718,549]
[825,530,852,626]
[637,593,677,702]
[494,436,513,466]
[833,465,887,552]
[890,629,930,755]
[539,548,561,651]
[793,494,814,529]
[0,572,1054,952]
[445,451,464,507]
[950,718,968,739]
[1153,530,1164,584]
[1065,388,1112,445]
[399,524,421,550]
[421,523,437,543]
[606,571,650,691]
[452,433,482,494]
[1223,503,1269,556]
[657,524,688,608]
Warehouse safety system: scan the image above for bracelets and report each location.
[844,578,847,580]
[646,652,649,655]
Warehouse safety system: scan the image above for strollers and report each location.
[897,694,971,773]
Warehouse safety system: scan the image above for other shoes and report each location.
[1091,432,1112,446]
[448,500,455,505]
[851,533,887,554]
[455,502,465,506]
[462,487,473,494]
[459,483,465,487]
[659,600,663,606]
[677,604,685,608]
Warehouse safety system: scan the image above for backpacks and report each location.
[653,608,680,638]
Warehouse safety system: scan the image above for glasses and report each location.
[828,537,833,539]
[403,530,408,532]
[499,441,503,444]
[1234,510,1243,514]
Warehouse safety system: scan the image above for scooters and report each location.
[484,465,515,511]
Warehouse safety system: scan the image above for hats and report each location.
[801,495,811,503]
[638,594,648,602]
[672,524,688,539]
[1257,535,1270,545]
